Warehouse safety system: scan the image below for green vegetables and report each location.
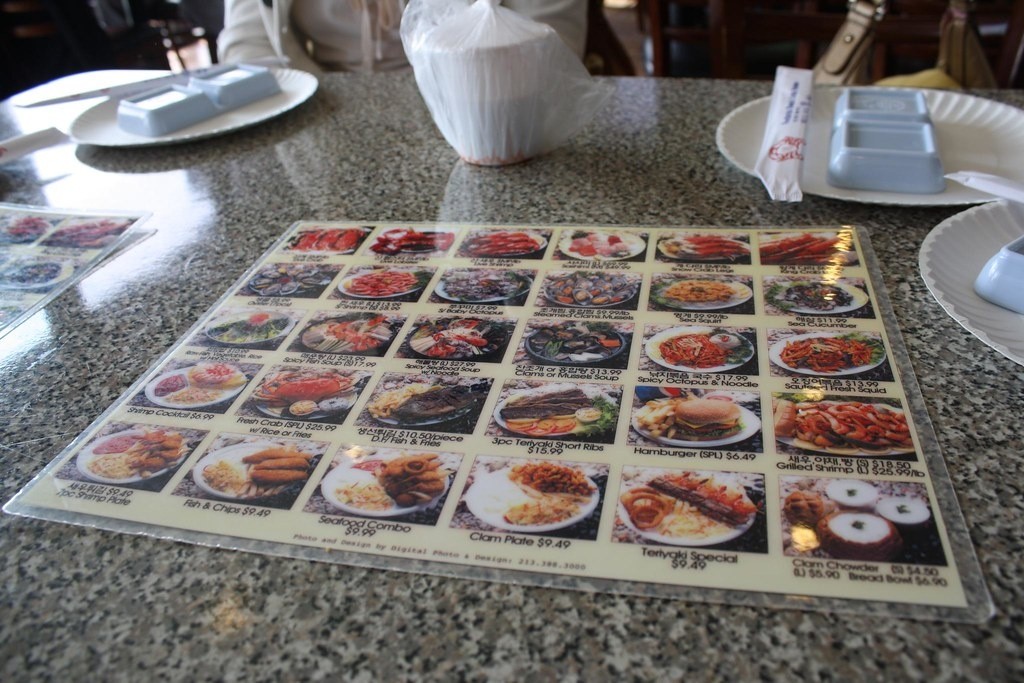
[572,394,618,438]
[830,331,884,364]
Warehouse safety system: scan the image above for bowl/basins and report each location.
[427,99,554,166]
[414,21,556,99]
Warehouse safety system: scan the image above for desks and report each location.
[0,68,1024,683]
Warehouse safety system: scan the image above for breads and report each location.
[784,491,824,527]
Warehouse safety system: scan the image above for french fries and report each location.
[636,396,686,439]
[368,383,442,418]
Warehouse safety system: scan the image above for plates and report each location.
[68,68,319,146]
[715,87,1024,204]
[919,201,1024,367]
[77,226,915,546]
[0,261,74,289]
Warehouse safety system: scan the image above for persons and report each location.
[0,0,228,101]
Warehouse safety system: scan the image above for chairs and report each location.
[0,0,1024,89]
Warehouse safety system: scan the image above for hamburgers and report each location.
[673,399,740,442]
